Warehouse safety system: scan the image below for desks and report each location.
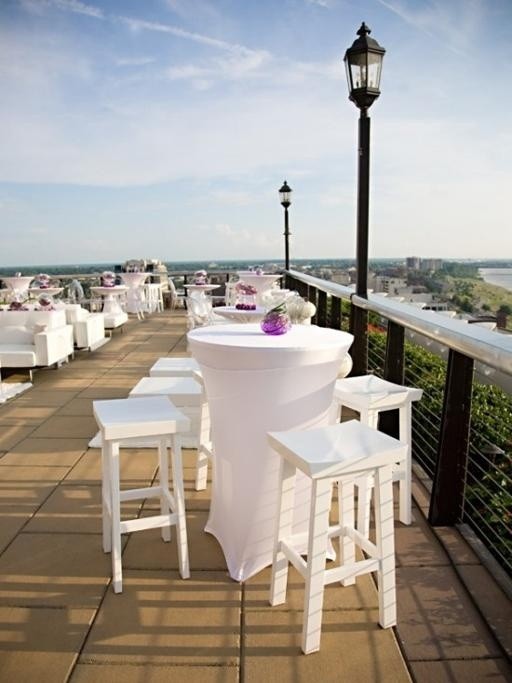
[186,321,357,582]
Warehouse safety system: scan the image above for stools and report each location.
[130,373,211,490]
[334,374,423,527]
[90,394,195,593]
[191,370,213,491]
[265,418,410,655]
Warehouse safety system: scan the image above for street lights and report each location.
[277,179,295,267]
[342,21,387,294]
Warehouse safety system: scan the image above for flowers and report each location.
[260,302,293,337]
